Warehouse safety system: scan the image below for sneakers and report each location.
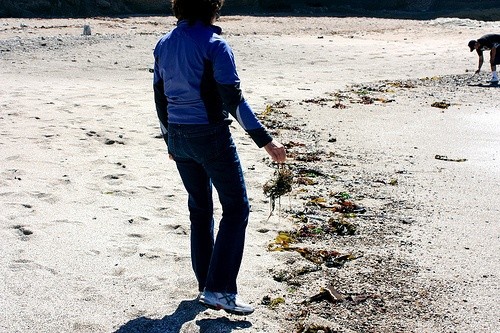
[197,287,207,304]
[199,287,255,315]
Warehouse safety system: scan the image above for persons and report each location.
[152,0,287,316]
[469,33,500,75]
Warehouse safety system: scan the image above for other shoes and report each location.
[486,76,499,82]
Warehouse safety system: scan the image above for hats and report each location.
[468,40,476,52]
